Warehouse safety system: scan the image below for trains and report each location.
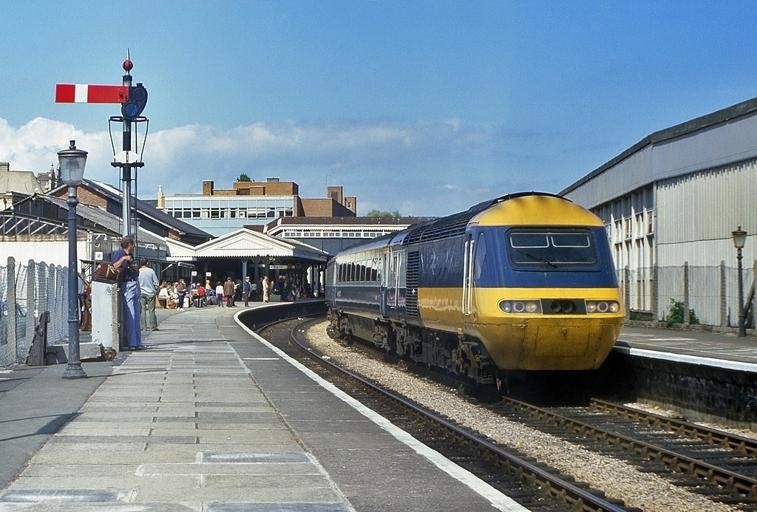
[324,191,627,401]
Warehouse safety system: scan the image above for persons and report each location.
[108,235,144,351]
[156,275,304,312]
[138,257,160,331]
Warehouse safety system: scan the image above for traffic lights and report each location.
[121,83,147,121]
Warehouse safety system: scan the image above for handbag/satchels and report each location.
[93,263,123,281]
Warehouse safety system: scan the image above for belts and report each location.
[123,279,136,282]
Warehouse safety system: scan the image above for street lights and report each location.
[58,141,88,381]
[731,225,747,337]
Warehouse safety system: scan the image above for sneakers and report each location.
[129,346,136,351]
[137,346,142,350]
[152,328,159,331]
